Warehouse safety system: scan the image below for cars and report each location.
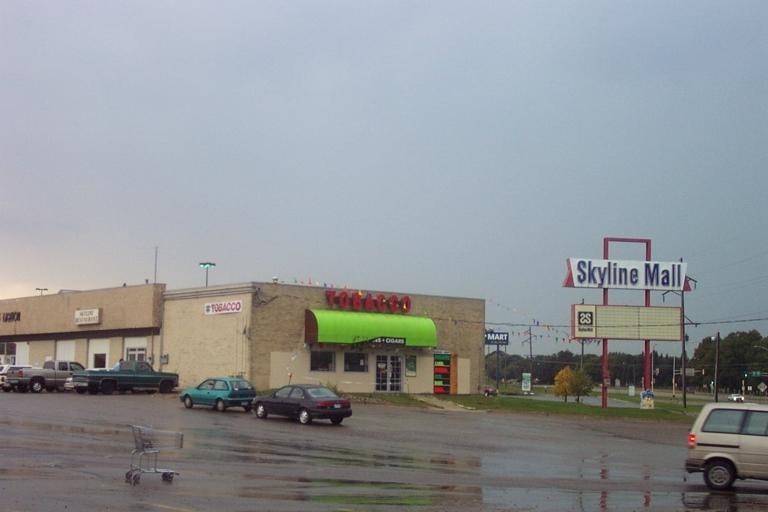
[178,376,256,413]
[480,385,498,397]
[250,383,353,426]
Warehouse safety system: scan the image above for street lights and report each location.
[752,345,767,351]
[651,343,658,393]
[710,330,721,404]
[198,261,217,288]
[35,288,48,295]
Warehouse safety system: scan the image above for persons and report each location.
[112,358,124,371]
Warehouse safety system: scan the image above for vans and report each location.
[683,401,767,491]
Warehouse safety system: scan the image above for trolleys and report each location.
[123,422,184,484]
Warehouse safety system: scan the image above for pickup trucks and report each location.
[0,358,179,395]
[727,394,744,402]
